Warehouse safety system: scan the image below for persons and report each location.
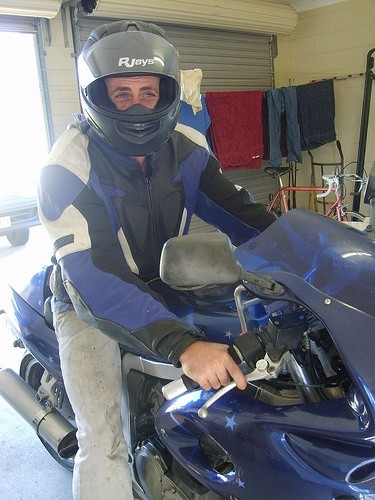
[35,19,285,500]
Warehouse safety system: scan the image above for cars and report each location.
[0,191,43,248]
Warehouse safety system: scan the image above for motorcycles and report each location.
[0,208,374,500]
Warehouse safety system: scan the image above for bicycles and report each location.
[260,161,374,245]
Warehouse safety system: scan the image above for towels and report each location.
[297,79,337,151]
[177,93,211,134]
[205,90,264,170]
[179,68,203,115]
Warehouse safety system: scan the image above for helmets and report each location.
[76,20,181,157]
